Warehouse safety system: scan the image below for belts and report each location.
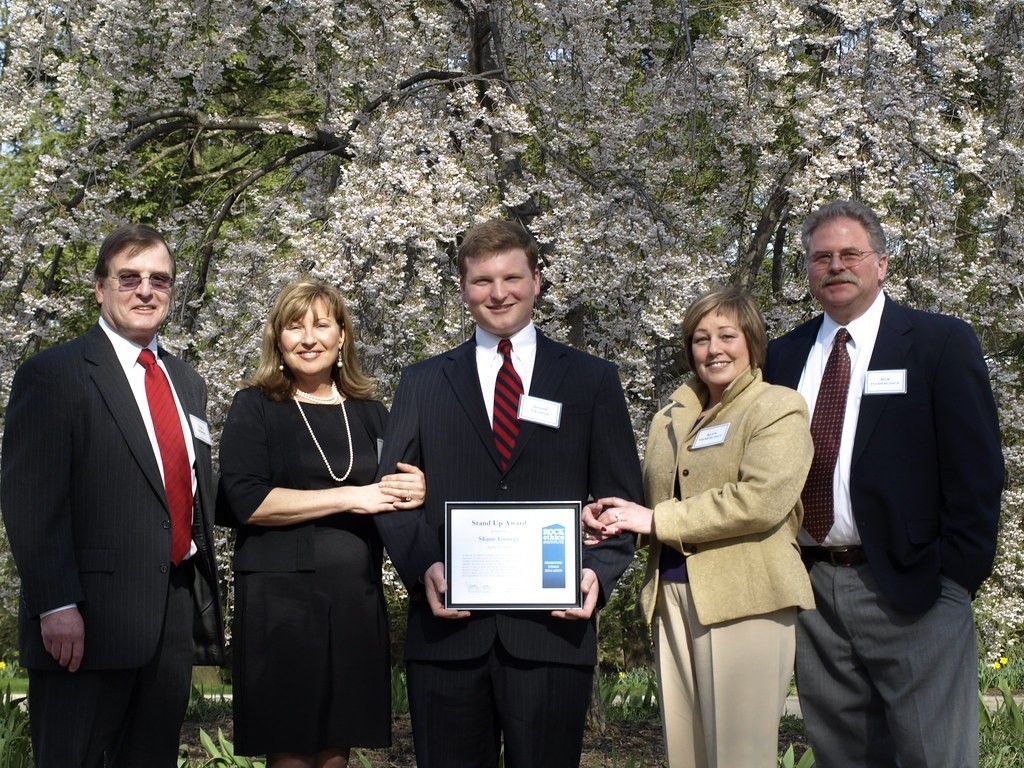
[803,546,867,567]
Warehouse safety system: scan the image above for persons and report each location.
[373,220,645,768]
[582,287,817,768]
[214,281,426,768]
[0,223,227,768]
[763,201,1005,768]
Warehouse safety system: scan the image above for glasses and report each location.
[118,272,173,290]
[805,249,875,265]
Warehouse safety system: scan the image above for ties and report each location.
[493,339,525,473]
[137,349,192,566]
[801,329,852,545]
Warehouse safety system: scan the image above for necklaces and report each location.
[292,379,353,481]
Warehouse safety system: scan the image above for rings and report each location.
[615,514,620,521]
[585,533,589,540]
[406,490,412,501]
